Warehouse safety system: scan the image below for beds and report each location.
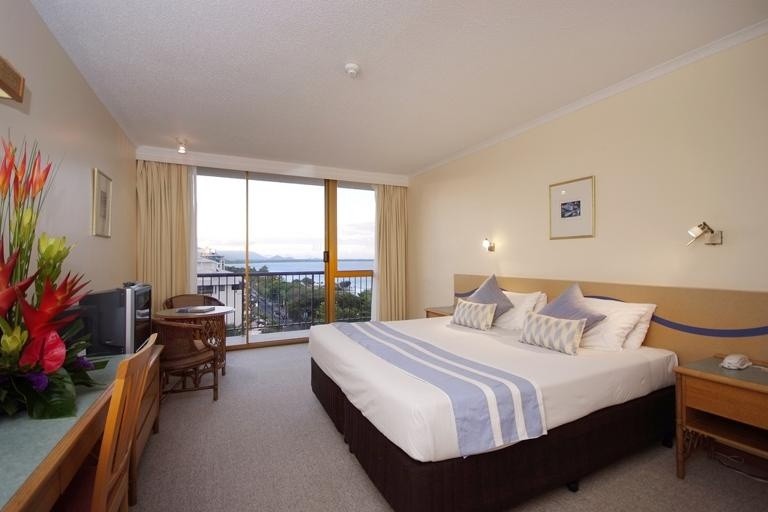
[309,314,680,512]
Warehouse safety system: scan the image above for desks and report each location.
[0,354,137,511]
[155,306,235,376]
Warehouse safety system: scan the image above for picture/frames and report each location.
[92,166,112,240]
[549,176,596,239]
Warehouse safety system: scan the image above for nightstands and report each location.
[424,306,456,317]
[675,353,764,479]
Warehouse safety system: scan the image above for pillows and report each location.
[538,283,606,333]
[450,298,496,332]
[582,304,649,350]
[519,310,587,355]
[584,298,658,349]
[534,293,547,313]
[466,274,514,323]
[495,290,542,330]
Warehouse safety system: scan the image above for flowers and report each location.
[0,129,110,420]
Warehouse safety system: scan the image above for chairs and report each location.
[57,332,159,511]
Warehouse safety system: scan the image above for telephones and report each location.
[720,353,753,371]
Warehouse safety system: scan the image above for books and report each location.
[175,305,215,313]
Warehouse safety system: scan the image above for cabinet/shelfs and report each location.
[137,344,166,470]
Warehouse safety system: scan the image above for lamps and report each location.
[178,142,188,154]
[345,63,359,77]
[688,222,724,247]
[484,238,495,252]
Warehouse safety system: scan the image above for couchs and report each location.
[163,293,225,309]
[152,319,218,402]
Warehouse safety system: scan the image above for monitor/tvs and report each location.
[79,281,153,356]
